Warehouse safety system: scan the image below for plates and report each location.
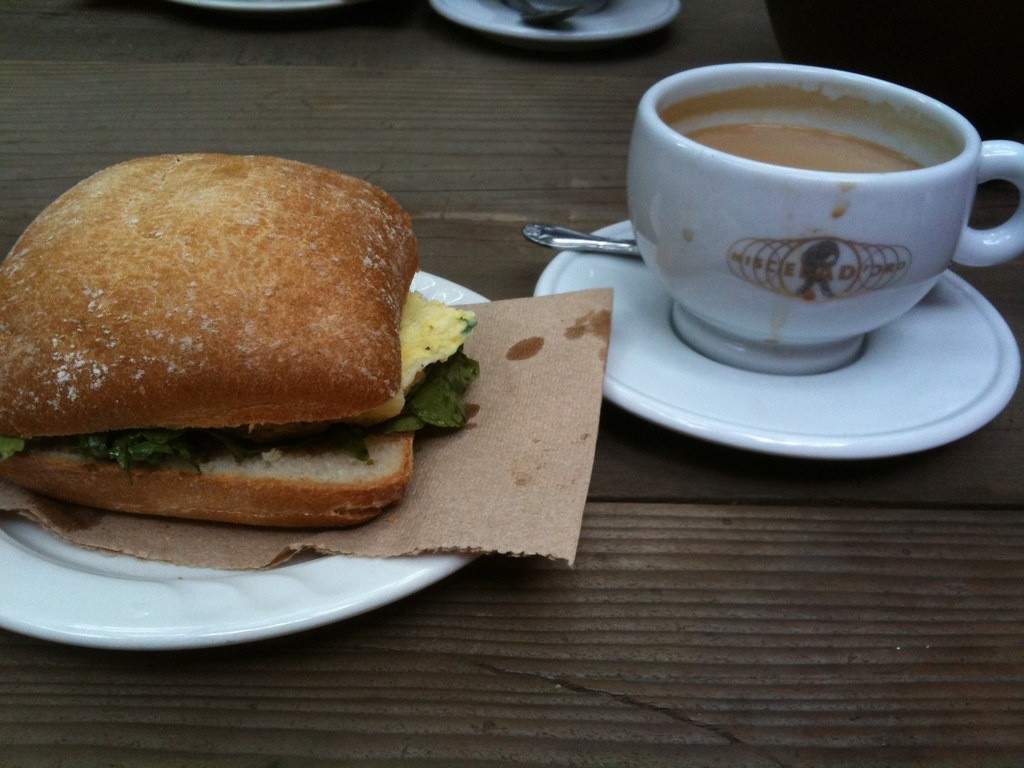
[431,0,681,40]
[0,272,491,652]
[533,216,1019,458]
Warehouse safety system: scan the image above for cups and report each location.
[626,60,1023,376]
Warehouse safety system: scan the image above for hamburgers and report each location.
[1,153,479,527]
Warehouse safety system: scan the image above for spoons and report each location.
[504,0,583,25]
